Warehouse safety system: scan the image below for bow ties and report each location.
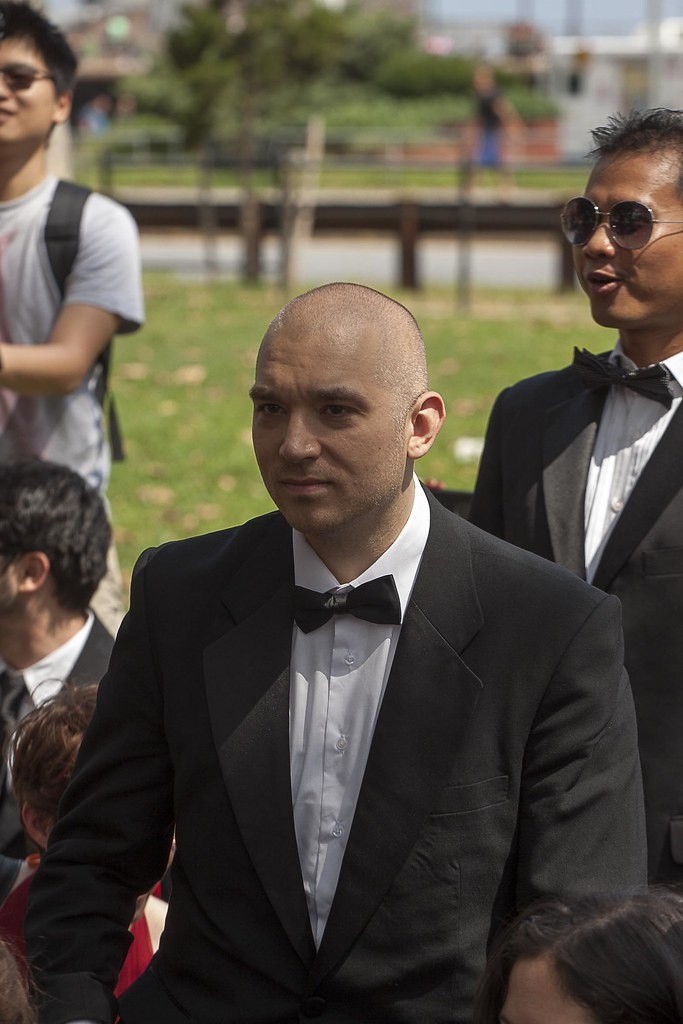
[572,346,672,410]
[295,574,402,635]
[0,674,25,726]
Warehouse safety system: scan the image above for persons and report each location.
[0,460,178,1024]
[0,0,144,641]
[25,284,649,1024]
[472,884,683,1024]
[471,110,683,885]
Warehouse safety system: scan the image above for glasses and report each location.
[0,65,52,90]
[560,196,683,250]
